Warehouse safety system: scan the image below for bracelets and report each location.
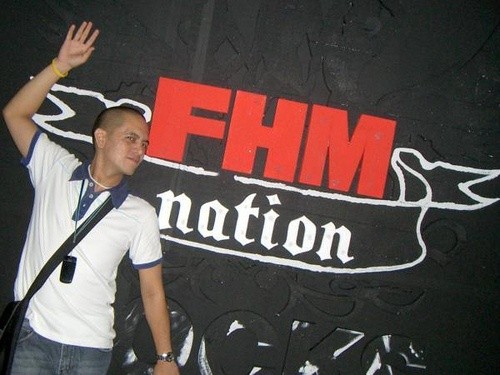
[51,57,68,77]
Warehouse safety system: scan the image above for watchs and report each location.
[155,352,175,362]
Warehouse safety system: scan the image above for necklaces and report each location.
[88,164,123,189]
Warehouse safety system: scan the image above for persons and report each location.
[2,21,180,375]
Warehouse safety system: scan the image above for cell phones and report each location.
[59,256,77,283]
